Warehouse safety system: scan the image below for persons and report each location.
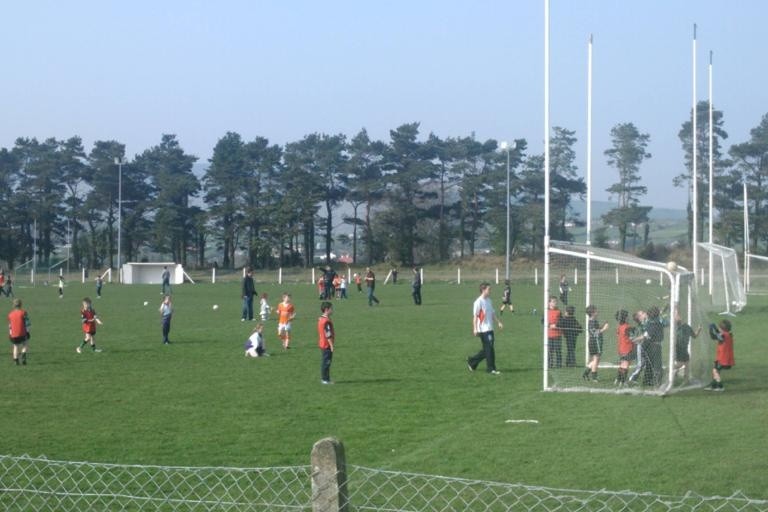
[239,267,297,358]
[317,302,335,385]
[315,264,380,307]
[6,299,32,365]
[5,275,14,297]
[465,282,504,375]
[391,267,400,283]
[0,273,6,296]
[57,276,65,298]
[704,319,736,392]
[159,266,173,295]
[160,296,174,344]
[76,297,104,353]
[540,274,670,389]
[411,268,422,305]
[499,280,515,318]
[95,275,103,299]
[671,306,703,389]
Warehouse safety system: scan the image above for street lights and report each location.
[497,140,518,286]
[111,154,125,268]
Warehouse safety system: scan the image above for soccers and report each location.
[212,304,218,311]
[667,261,678,272]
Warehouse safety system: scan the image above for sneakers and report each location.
[704,383,715,391]
[320,379,335,385]
[490,370,501,375]
[593,379,602,383]
[582,375,590,382]
[466,358,475,372]
[614,379,629,386]
[91,348,102,353]
[712,384,724,391]
[76,347,83,354]
